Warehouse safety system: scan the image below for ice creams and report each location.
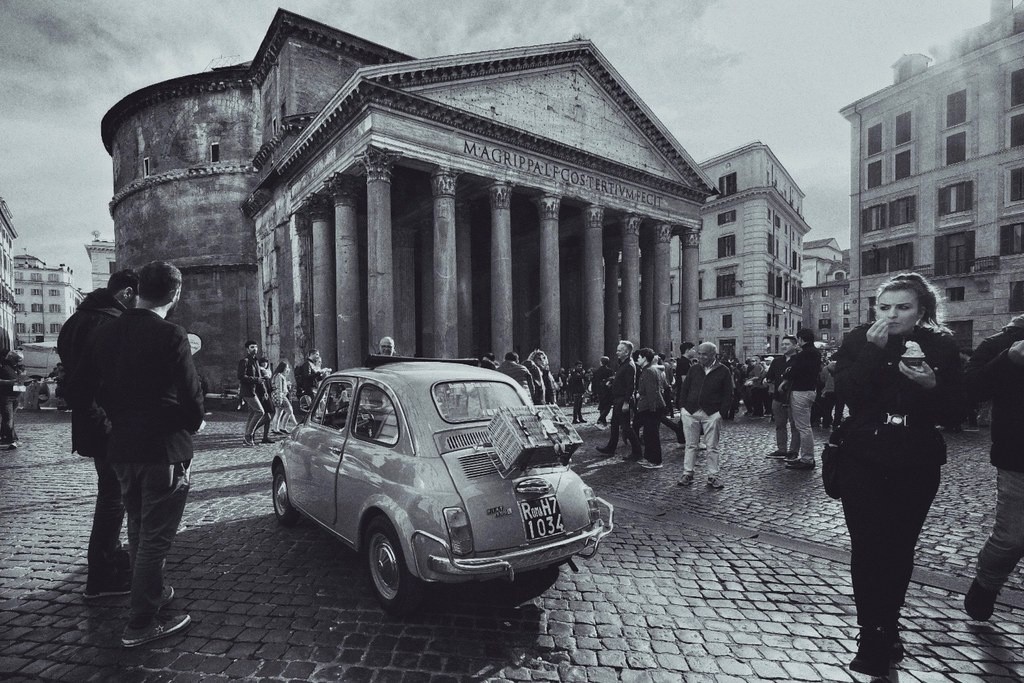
[901,341,925,358]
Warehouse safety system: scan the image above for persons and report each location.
[237,340,350,448]
[820,270,968,677]
[55,261,209,649]
[478,327,981,490]
[964,313,1024,623]
[0,349,68,450]
[363,336,400,368]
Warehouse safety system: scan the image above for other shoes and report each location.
[677,475,694,485]
[83,581,131,599]
[262,438,275,443]
[573,419,580,423]
[964,577,998,622]
[636,458,663,468]
[765,451,786,458]
[8,441,18,449]
[785,459,815,470]
[850,621,904,676]
[706,477,724,488]
[243,434,253,446]
[623,453,642,460]
[782,451,799,462]
[269,430,283,436]
[150,586,174,614]
[595,446,615,455]
[122,614,191,647]
[579,417,587,423]
[279,429,290,435]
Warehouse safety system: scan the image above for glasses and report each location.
[381,345,392,349]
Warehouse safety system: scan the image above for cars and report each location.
[270,356,616,617]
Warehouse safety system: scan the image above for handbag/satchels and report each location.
[622,390,648,417]
[262,393,273,412]
[271,389,283,407]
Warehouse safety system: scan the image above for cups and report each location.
[902,357,924,370]
